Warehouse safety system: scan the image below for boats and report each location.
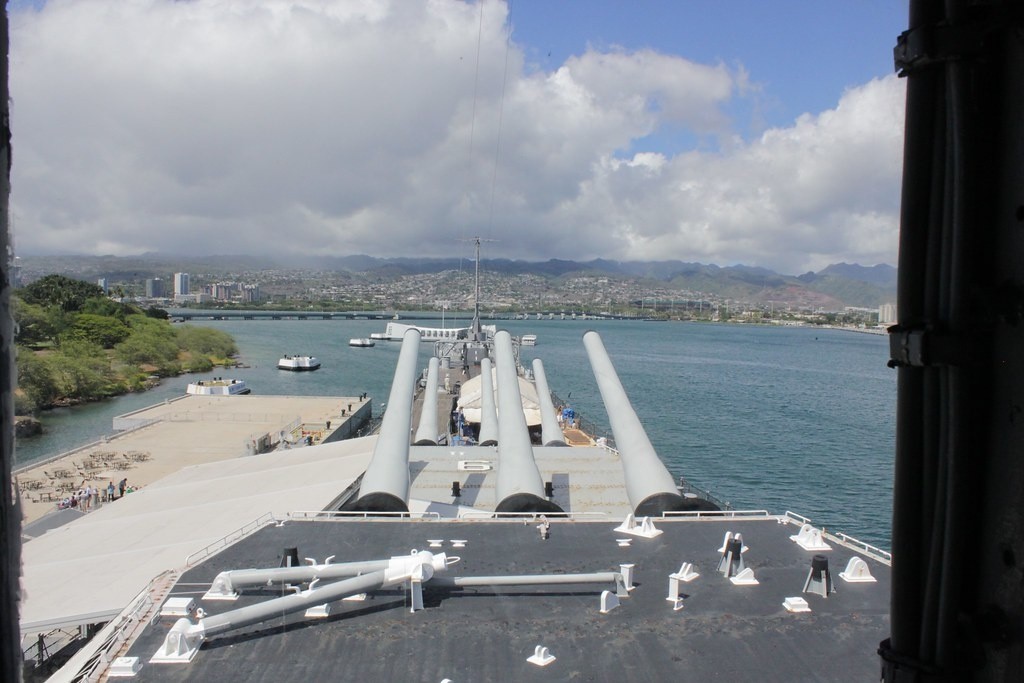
[187,378,251,397]
[371,333,393,341]
[384,235,500,344]
[349,337,374,348]
[277,355,322,372]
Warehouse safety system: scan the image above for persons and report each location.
[119,478,127,497]
[56,484,92,510]
[107,481,115,502]
